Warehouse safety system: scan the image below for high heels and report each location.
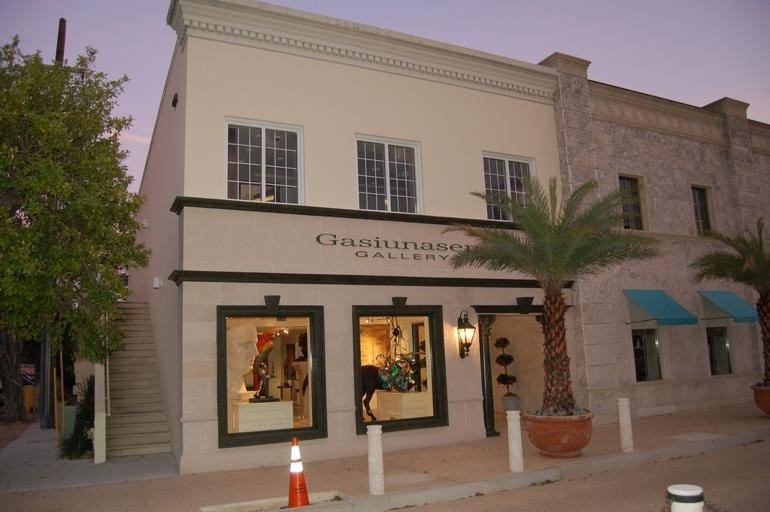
[280,436,309,509]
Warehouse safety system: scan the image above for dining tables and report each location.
[494,336,519,416]
[442,174,662,457]
[687,218,769,414]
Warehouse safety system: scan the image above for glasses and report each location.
[271,326,289,339]
[455,309,476,359]
[142,219,147,228]
[153,278,162,289]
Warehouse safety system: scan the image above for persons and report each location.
[227,323,262,401]
